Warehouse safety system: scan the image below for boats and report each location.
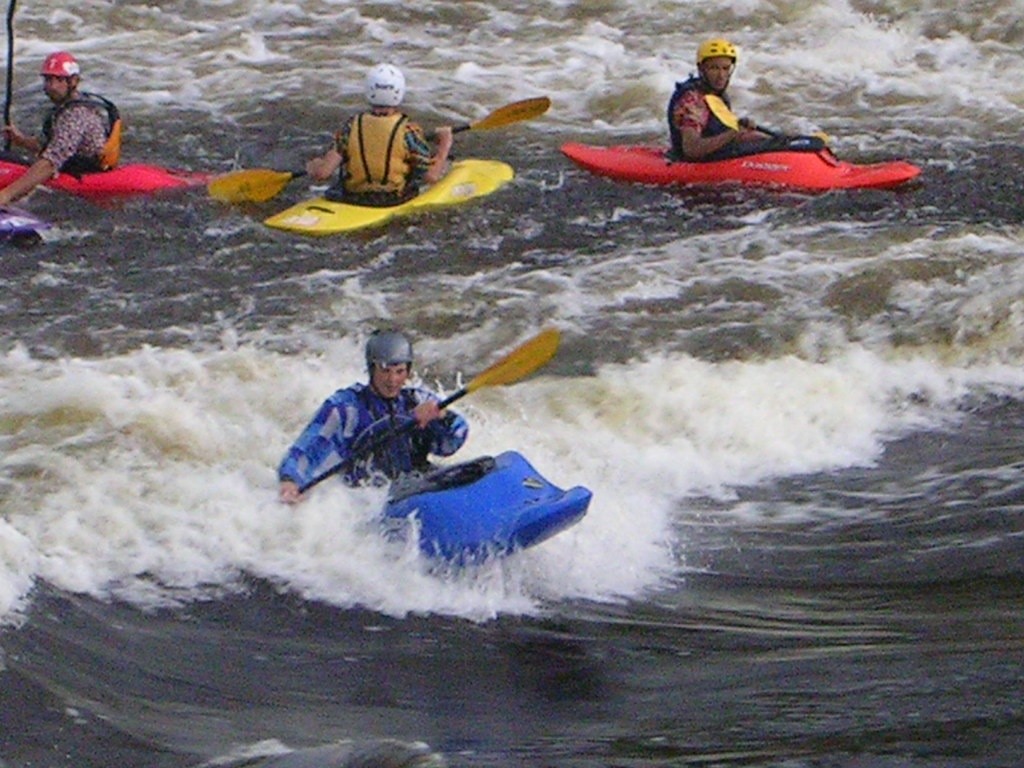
[0,157,221,197]
[377,450,593,571]
[261,156,514,236]
[561,140,925,195]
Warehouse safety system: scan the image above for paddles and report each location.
[703,92,830,144]
[297,327,565,496]
[207,96,553,204]
[4,0,20,155]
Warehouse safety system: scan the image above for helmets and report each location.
[365,330,413,369]
[365,63,405,106]
[39,52,80,77]
[696,39,737,65]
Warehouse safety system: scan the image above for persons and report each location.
[1,51,122,207]
[667,38,786,162]
[278,332,469,505]
[306,63,453,208]
